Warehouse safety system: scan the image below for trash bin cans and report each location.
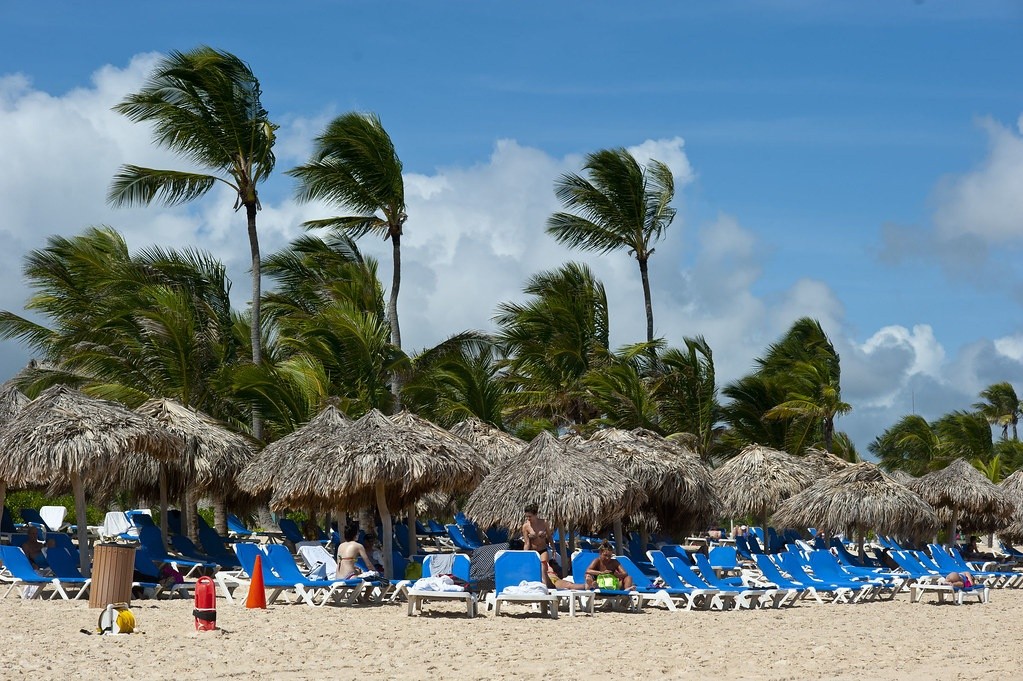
[88,542,137,610]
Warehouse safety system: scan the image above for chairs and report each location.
[0,504,1023,620]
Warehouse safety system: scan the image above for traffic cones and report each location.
[245,555,268,610]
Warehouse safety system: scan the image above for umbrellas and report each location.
[0,380,258,576]
[233,402,487,578]
[906,454,1023,550]
[444,417,716,577]
[711,441,940,559]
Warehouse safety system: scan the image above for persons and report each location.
[363,532,384,570]
[336,525,391,604]
[944,571,976,587]
[548,561,594,589]
[522,503,557,586]
[21,525,55,577]
[738,525,744,536]
[966,535,988,561]
[586,537,633,607]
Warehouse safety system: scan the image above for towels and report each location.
[503,579,549,595]
[410,575,464,593]
[39,506,68,531]
[104,512,132,538]
[297,546,379,581]
